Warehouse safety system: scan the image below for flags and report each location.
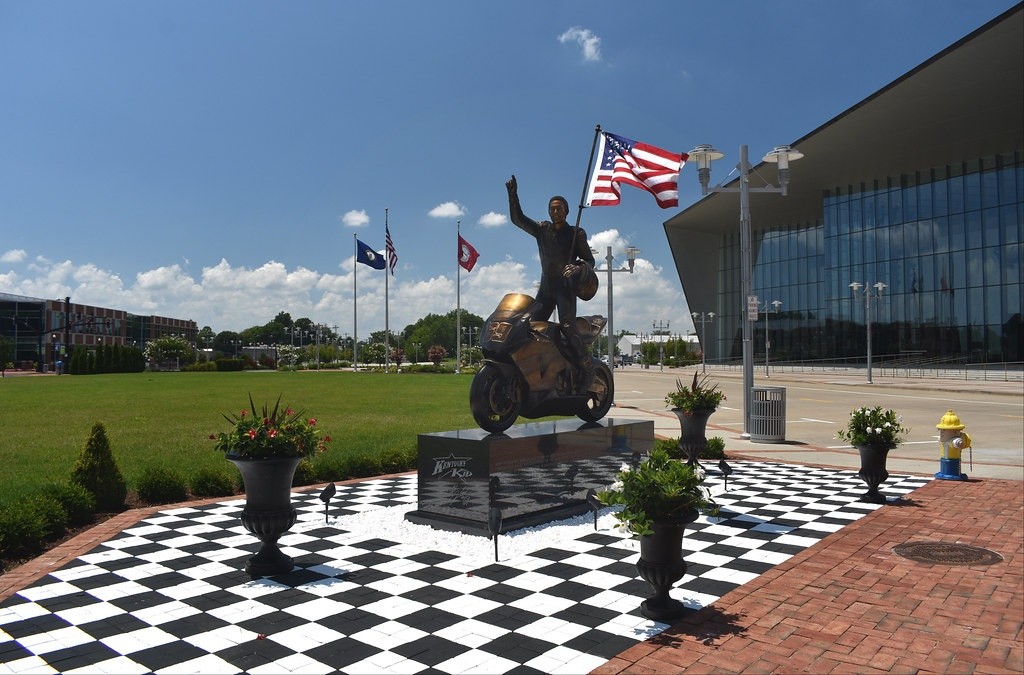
[353,237,386,270]
[385,223,399,277]
[456,231,480,272]
[583,128,690,207]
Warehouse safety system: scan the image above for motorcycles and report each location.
[467,292,611,435]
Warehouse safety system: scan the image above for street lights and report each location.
[849,282,889,385]
[756,300,784,378]
[692,311,716,375]
[683,145,807,440]
[652,320,671,373]
[586,244,642,404]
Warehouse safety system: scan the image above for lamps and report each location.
[631,451,641,465]
[319,482,336,502]
[718,459,733,475]
[587,488,604,510]
[488,508,502,535]
[566,466,578,479]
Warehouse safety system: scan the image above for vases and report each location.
[634,509,700,618]
[857,445,896,503]
[672,409,717,471]
[226,448,303,576]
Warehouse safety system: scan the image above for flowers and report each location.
[208,392,333,463]
[664,369,727,418]
[591,449,721,548]
[834,405,912,446]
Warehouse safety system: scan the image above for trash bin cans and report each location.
[750,386,787,443]
[56,361,63,376]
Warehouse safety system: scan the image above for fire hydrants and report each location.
[934,408,973,482]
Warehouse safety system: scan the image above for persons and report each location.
[505,174,599,395]
